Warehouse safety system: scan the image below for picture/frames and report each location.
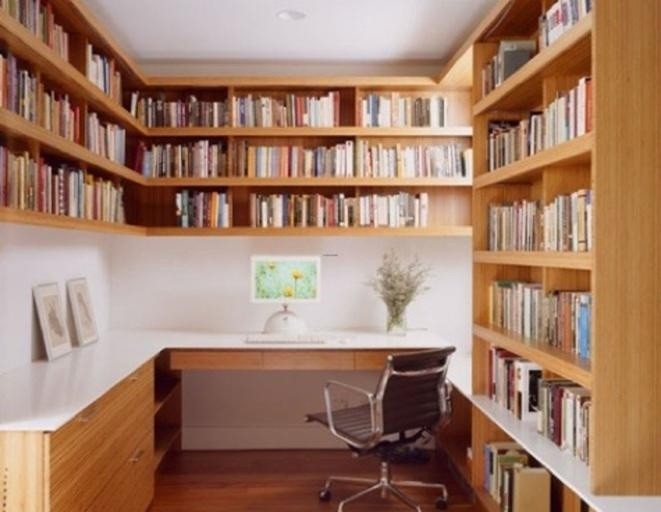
[32,275,103,361]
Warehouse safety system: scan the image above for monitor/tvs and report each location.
[251,254,322,332]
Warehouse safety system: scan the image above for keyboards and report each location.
[244,331,327,345]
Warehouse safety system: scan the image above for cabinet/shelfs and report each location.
[443,0,661,511]
[0,0,474,238]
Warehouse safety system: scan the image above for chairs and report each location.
[303,343,455,511]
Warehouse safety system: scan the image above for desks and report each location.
[0,329,458,512]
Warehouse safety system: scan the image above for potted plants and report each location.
[366,246,435,338]
